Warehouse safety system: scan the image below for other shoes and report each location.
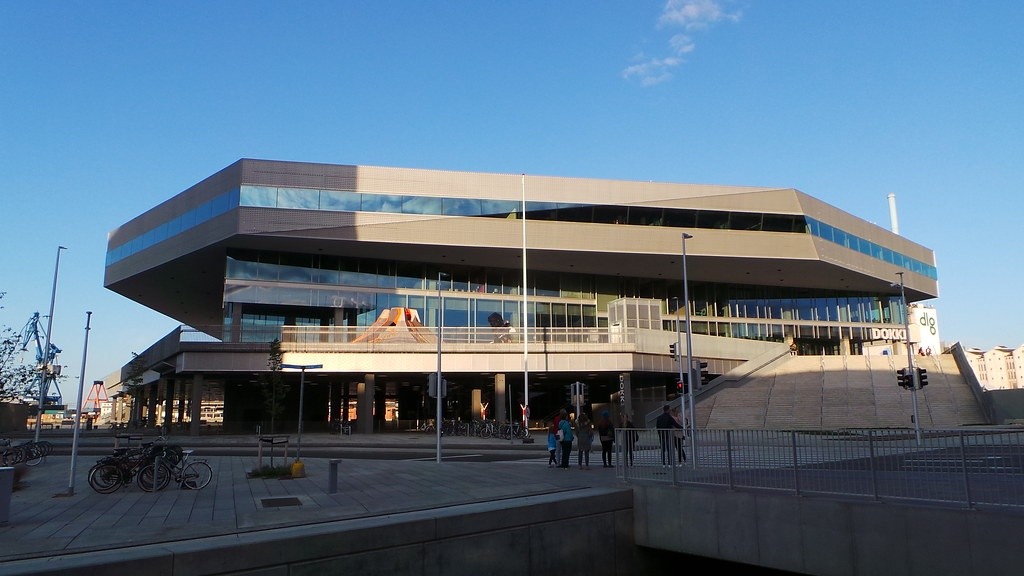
[609,465,614,468]
[663,463,687,468]
[603,464,608,468]
[548,463,568,471]
[578,464,582,470]
[585,466,592,470]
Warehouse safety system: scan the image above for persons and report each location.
[790,343,798,356]
[619,414,638,466]
[598,410,615,468]
[553,409,574,468]
[918,347,925,356]
[547,426,557,467]
[501,320,512,343]
[575,414,594,470]
[926,346,931,356]
[656,405,687,465]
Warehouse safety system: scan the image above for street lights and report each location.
[436,271,450,462]
[32,244,69,456]
[890,270,922,446]
[66,310,94,495]
[681,232,698,470]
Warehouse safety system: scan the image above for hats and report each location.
[601,410,609,417]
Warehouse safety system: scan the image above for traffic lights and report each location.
[897,367,910,391]
[669,342,677,362]
[676,381,684,395]
[904,374,915,388]
[913,367,929,390]
[695,359,709,390]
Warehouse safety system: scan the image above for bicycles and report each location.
[420,416,531,441]
[0,436,54,467]
[86,432,214,495]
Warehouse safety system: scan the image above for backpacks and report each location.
[555,421,566,441]
